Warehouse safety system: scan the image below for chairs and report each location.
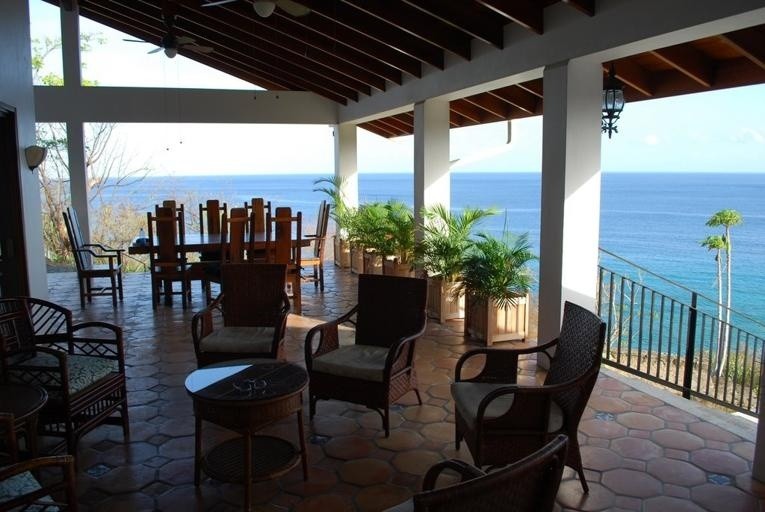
[451,301,606,493]
[62,207,125,307]
[305,274,429,438]
[147,198,330,311]
[0,455,78,512]
[383,434,569,512]
[0,296,129,472]
[192,263,291,368]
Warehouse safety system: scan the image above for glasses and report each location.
[233,379,267,392]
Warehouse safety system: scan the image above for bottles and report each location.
[139,228,146,246]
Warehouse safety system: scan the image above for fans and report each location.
[200,0,312,17]
[123,19,213,55]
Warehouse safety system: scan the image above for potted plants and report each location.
[313,175,539,347]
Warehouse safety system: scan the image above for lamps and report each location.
[601,63,624,138]
[25,146,48,171]
[253,1,276,17]
[164,40,177,58]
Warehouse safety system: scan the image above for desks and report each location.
[129,233,309,306]
